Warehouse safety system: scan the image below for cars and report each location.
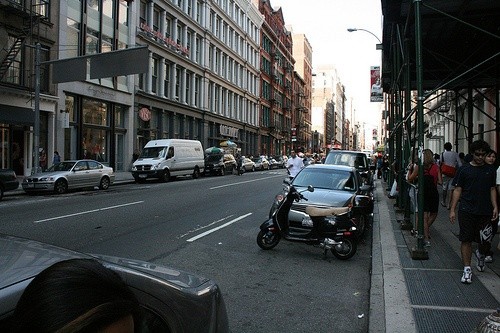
[203,153,237,176]
[1,232,231,333]
[266,155,288,170]
[324,149,375,185]
[22,159,116,195]
[235,156,256,171]
[268,163,370,239]
[251,158,269,170]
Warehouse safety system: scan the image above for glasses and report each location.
[474,152,486,157]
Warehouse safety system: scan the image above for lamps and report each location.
[60,96,74,113]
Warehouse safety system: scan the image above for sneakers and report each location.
[476,249,486,272]
[461,267,473,283]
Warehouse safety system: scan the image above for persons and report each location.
[496,164,500,194]
[449,140,498,283]
[235,153,243,176]
[54,151,60,165]
[38,147,47,172]
[478,150,498,264]
[286,149,326,182]
[372,141,472,248]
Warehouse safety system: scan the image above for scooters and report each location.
[256,177,360,262]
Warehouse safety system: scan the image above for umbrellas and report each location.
[204,146,224,154]
[219,140,238,148]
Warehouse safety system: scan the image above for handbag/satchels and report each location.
[391,182,397,196]
[441,163,456,177]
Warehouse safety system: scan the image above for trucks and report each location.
[130,138,205,183]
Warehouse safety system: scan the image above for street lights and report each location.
[347,27,388,153]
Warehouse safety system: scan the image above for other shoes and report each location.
[440,200,447,207]
[425,241,431,246]
